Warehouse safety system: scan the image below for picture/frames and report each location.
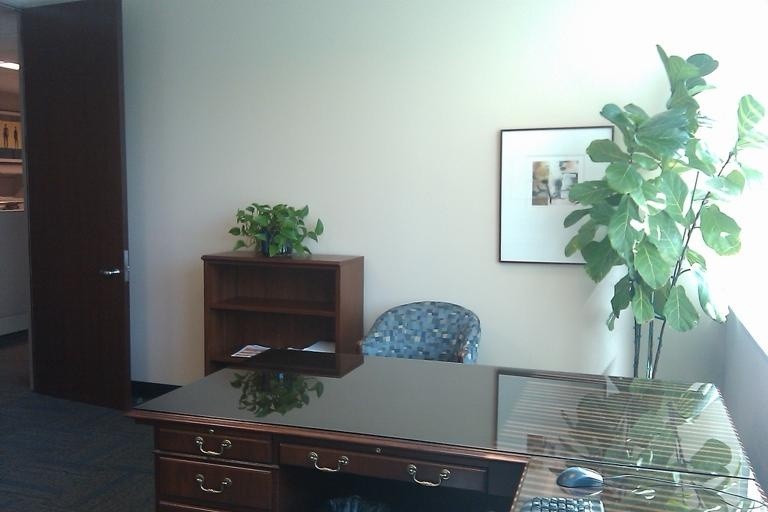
[0,111,23,163]
[498,125,615,265]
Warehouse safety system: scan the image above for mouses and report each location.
[556,467,604,488]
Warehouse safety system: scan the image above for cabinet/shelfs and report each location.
[200,250,364,380]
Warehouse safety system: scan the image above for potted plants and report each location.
[228,202,324,258]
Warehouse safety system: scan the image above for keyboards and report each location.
[520,496,606,512]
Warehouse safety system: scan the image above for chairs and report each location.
[359,300,481,364]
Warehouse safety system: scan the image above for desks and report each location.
[124,343,762,512]
